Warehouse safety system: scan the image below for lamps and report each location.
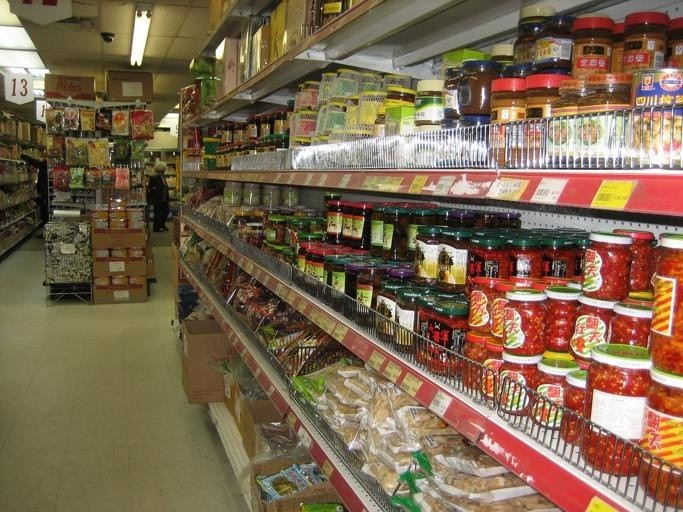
[129,3,152,67]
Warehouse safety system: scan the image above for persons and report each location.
[146,161,170,232]
[20,148,54,240]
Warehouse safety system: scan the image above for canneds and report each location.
[216,179,683,509]
[214,3,683,170]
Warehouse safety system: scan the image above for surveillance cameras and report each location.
[101,32,115,43]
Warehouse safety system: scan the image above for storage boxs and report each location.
[91,220,148,304]
[44,72,95,99]
[103,70,153,104]
[225,371,349,512]
[179,317,235,404]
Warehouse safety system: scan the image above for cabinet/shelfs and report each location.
[143,148,180,221]
[42,98,157,297]
[1,97,48,256]
[171,0,683,511]
